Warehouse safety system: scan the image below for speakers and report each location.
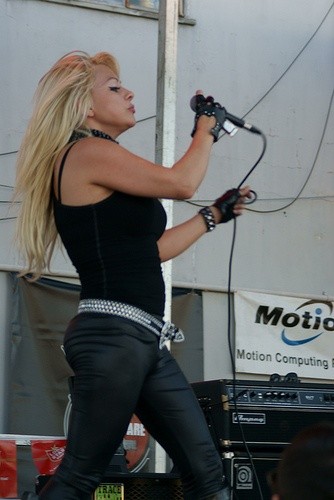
[37,471,184,500]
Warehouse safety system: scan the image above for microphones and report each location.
[190,95,263,135]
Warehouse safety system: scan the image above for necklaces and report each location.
[74,128,119,144]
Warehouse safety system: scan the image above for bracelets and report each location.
[198,206,216,232]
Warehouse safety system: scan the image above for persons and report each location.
[283,423,334,500]
[23,47,253,500]
